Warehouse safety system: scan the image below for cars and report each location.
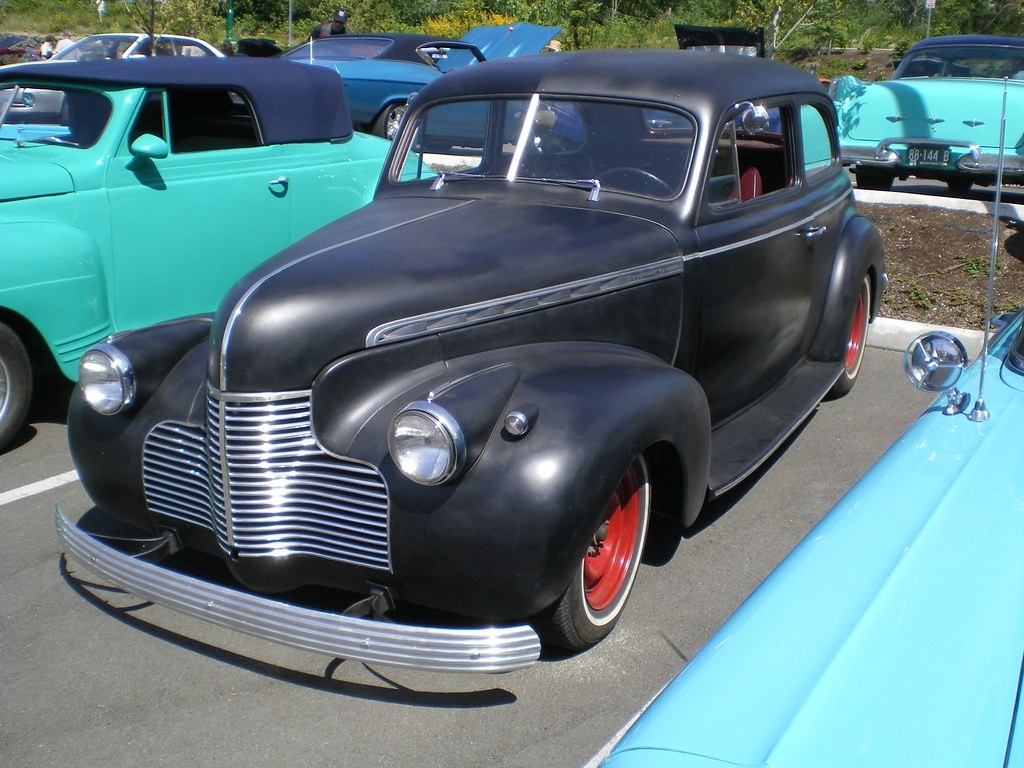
[585,213,1024,768]
[0,21,782,151]
[54,47,884,696]
[0,55,438,456]
[838,35,1023,190]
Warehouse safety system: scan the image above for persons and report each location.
[187,27,197,38]
[222,38,233,57]
[52,29,82,60]
[307,9,352,57]
[40,35,54,61]
[545,39,562,53]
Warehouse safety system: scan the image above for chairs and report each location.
[534,153,593,184]
[714,155,778,206]
